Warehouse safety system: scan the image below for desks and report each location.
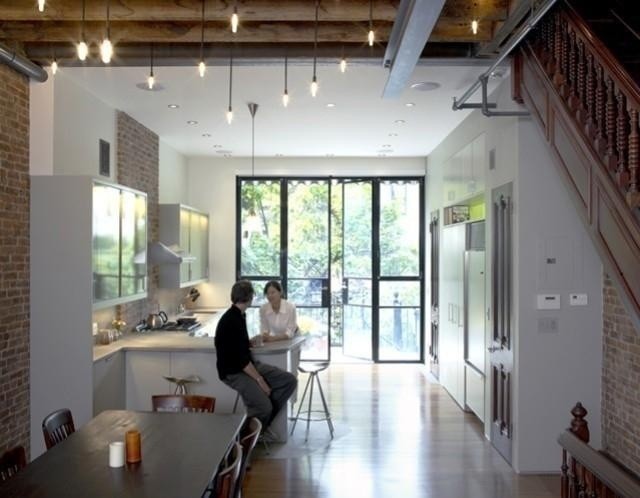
[121,332,309,445]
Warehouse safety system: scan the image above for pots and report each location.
[177,318,197,326]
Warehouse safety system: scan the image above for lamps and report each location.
[470,0,480,35]
[146,0,378,127]
[245,104,260,230]
[71,1,91,64]
[96,1,116,66]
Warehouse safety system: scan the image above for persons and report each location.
[257,280,298,340]
[214,279,299,444]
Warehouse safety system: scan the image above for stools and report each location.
[291,362,335,444]
[164,375,202,394]
[234,385,280,456]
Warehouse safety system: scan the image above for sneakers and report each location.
[265,428,279,440]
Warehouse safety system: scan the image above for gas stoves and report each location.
[143,322,201,331]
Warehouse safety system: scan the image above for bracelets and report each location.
[255,375,264,383]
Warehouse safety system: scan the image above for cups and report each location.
[125,428,142,465]
[108,442,126,469]
[97,329,121,344]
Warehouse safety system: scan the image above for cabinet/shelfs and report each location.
[28,345,125,422]
[29,173,149,312]
[438,120,520,414]
[158,203,209,289]
[125,349,233,408]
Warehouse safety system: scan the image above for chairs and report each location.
[42,407,75,452]
[0,445,28,482]
[237,416,262,497]
[149,395,218,412]
[203,439,244,497]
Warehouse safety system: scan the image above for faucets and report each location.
[177,291,199,314]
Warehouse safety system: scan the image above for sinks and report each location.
[185,310,219,316]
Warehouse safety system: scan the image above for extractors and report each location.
[148,240,197,265]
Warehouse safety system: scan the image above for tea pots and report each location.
[146,311,169,330]
